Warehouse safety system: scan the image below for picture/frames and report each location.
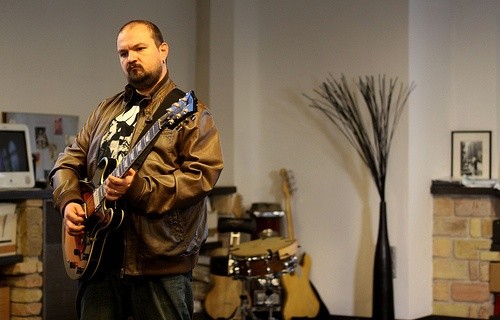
[452,131,492,181]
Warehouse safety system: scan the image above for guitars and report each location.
[62,89,200,280]
[205,275,241,319]
[281,170,321,320]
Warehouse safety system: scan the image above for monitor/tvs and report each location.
[0,125,36,189]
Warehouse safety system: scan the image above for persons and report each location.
[49,20,223,320]
[0,136,27,173]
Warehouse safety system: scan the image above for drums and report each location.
[218,216,257,249]
[208,186,239,214]
[228,237,298,275]
[249,201,287,238]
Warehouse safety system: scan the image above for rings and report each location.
[115,190,116,196]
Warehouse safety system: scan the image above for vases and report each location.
[372,202,395,320]
